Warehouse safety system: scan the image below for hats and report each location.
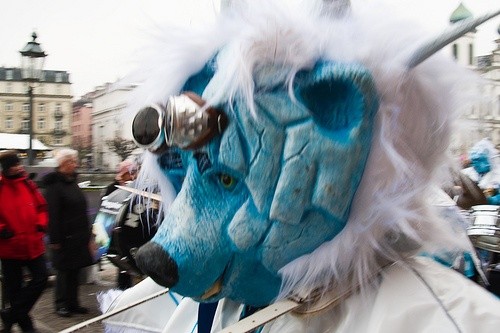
[0,150,20,166]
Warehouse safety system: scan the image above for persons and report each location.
[42,147,93,318]
[88,131,500,331]
[0,150,49,333]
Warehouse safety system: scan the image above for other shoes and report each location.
[72,306,89,315]
[1,308,13,333]
[57,307,70,317]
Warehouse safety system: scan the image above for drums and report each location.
[466,204,500,254]
[461,166,484,203]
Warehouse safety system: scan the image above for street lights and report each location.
[19,31,49,166]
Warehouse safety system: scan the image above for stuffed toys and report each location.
[101,0,497,332]
